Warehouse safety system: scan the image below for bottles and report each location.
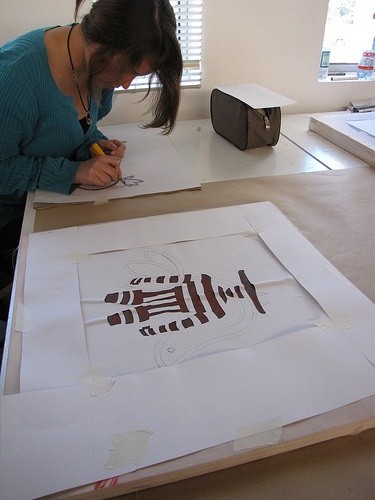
[355,12,375,78]
[318,18,332,81]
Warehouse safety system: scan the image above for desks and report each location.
[0,109,374,500]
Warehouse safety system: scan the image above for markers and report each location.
[331,75,359,80]
[93,142,128,186]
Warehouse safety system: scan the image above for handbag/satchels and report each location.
[210,89,281,151]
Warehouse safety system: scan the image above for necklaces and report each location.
[67,22,95,126]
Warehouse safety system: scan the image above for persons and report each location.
[0,0,184,290]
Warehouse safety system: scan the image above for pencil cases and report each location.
[209,87,282,151]
